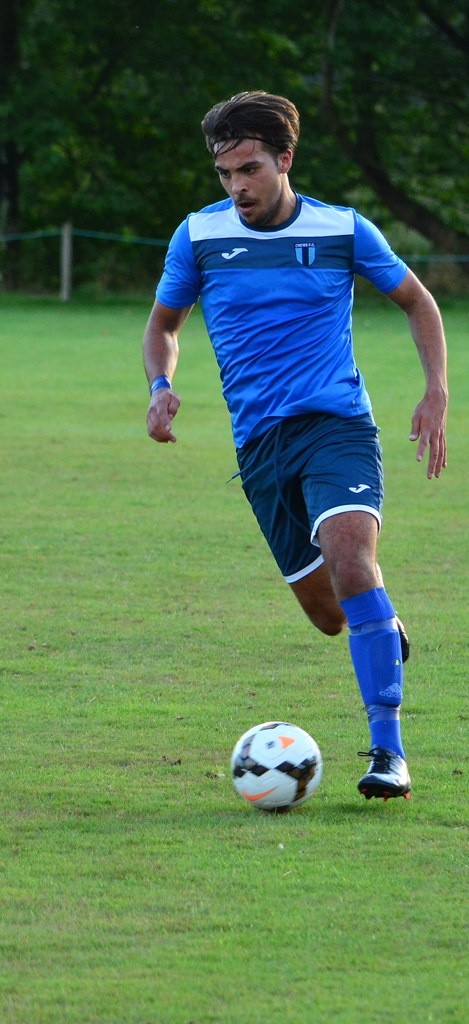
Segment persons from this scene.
[143,93,447,803]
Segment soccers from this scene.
[228,720,324,813]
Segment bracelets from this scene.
[149,375,171,396]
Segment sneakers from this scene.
[356,745,412,803]
[375,561,410,663]
